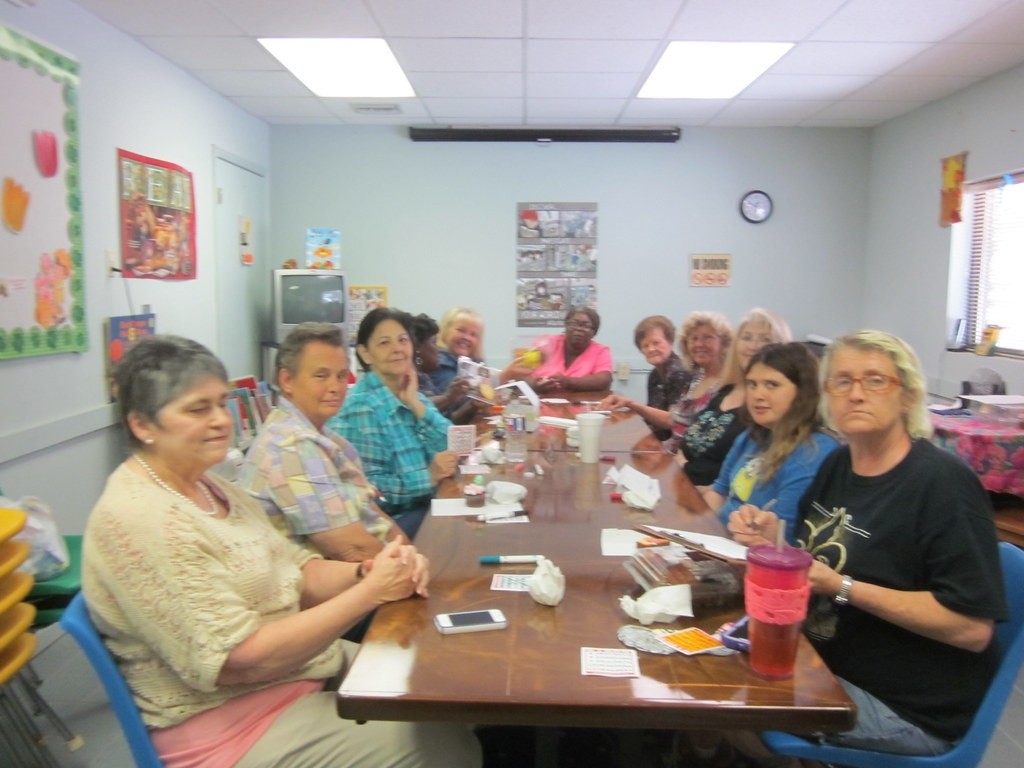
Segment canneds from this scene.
[976,325,1001,356]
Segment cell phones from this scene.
[722,614,750,652]
[433,609,507,634]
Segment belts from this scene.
[382,494,431,516]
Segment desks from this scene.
[929,403,1024,499]
[336,389,858,768]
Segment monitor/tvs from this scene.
[270,270,349,345]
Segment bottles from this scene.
[504,394,528,461]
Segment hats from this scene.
[411,313,440,344]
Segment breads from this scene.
[636,536,670,548]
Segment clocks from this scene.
[741,190,773,224]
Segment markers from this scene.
[477,510,528,522]
[572,400,600,405]
[480,555,546,563]
[611,493,661,501]
[575,452,615,461]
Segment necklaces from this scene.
[134,453,220,515]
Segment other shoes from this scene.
[654,737,735,768]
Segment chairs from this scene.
[762,540,1023,768]
[0,506,165,768]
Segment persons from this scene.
[512,304,614,393]
[702,340,843,545]
[80,332,483,768]
[592,310,733,457]
[413,313,502,425]
[236,321,410,562]
[634,314,697,440]
[322,307,461,542]
[435,306,533,392]
[675,307,793,493]
[681,328,1008,768]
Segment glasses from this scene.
[823,373,905,395]
[564,319,593,332]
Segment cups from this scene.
[744,543,813,681]
[576,412,606,464]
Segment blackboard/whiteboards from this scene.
[0,22,90,358]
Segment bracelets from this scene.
[355,563,365,584]
[831,575,852,606]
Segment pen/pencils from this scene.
[750,498,777,530]
